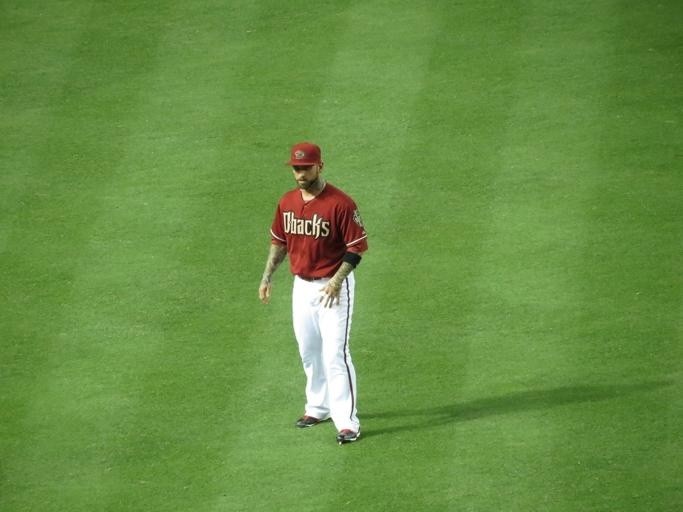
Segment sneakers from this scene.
[335,426,361,442]
[296,416,320,426]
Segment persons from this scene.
[258,141,368,445]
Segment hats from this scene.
[285,142,322,167]
